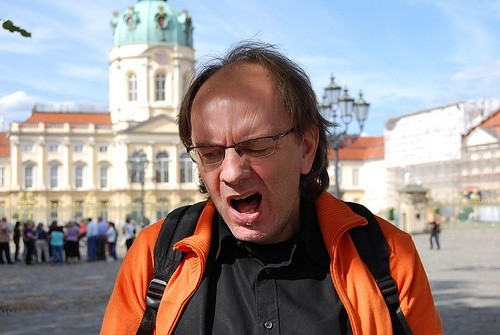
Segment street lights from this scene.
[317,74,370,201]
[126,148,149,228]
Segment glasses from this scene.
[187,125,299,167]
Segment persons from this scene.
[100,41,443,335]
[0,215,137,267]
[426,213,442,251]
